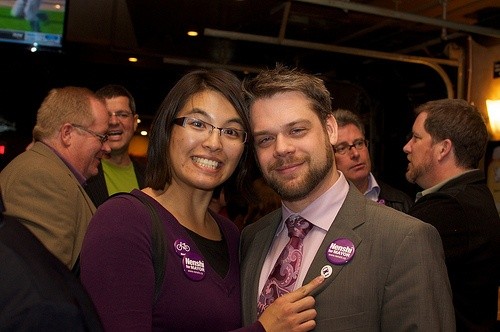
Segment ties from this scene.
[256,214,314,320]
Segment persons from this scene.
[332,98,500,332]
[237,62,456,332]
[79,70,324,332]
[0,81,279,332]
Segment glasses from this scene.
[60,123,108,144]
[334,139,369,154]
[173,116,248,144]
[108,111,134,119]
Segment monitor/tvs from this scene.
[0,0,69,51]
